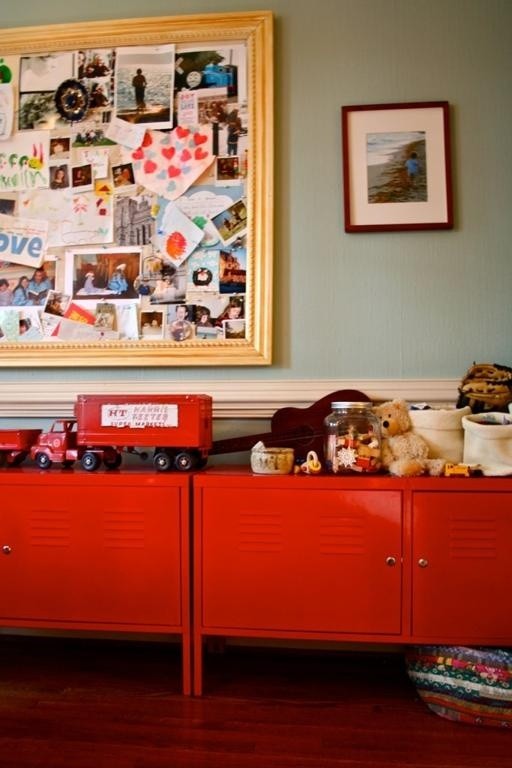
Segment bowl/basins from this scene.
[250,446,294,475]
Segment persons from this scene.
[204,100,241,155]
[142,300,245,341]
[0,267,53,336]
[405,152,419,187]
[51,169,66,190]
[79,52,147,114]
[51,140,69,160]
[74,169,86,186]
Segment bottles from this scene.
[323,401,383,474]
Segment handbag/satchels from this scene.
[403,644,512,731]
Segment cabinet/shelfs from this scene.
[1,460,512,696]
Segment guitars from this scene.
[209,389,374,463]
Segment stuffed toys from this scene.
[371,399,446,477]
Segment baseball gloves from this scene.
[458,364,511,406]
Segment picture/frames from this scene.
[0,3,280,369]
[341,97,458,234]
